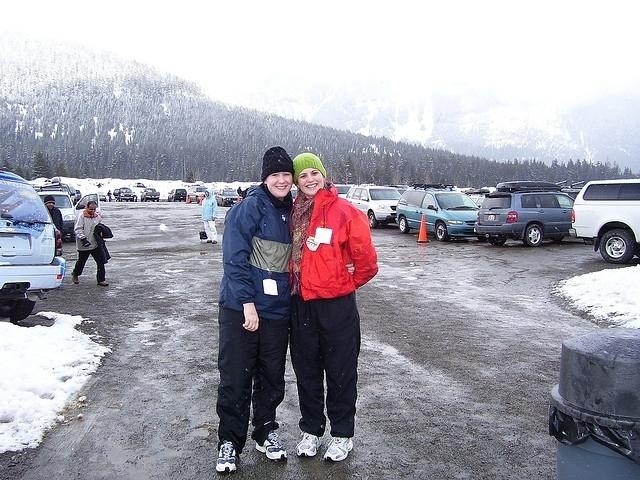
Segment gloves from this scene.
[80,238,90,247]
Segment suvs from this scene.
[0,167,107,325]
[113,187,137,201]
[568,178,640,266]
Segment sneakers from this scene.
[206,240,217,243]
[72,275,79,284]
[99,281,109,286]
[296,432,323,457]
[324,437,353,463]
[215,442,237,472]
[254,430,287,459]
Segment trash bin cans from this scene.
[548,328,640,480]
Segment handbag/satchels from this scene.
[200,232,207,238]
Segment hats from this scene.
[44,196,56,205]
[261,147,294,181]
[293,153,326,185]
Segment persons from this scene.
[71,200,110,287]
[213,146,295,474]
[288,153,379,461]
[201,187,219,244]
[43,195,63,232]
[106,189,113,202]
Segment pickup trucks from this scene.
[140,188,160,202]
